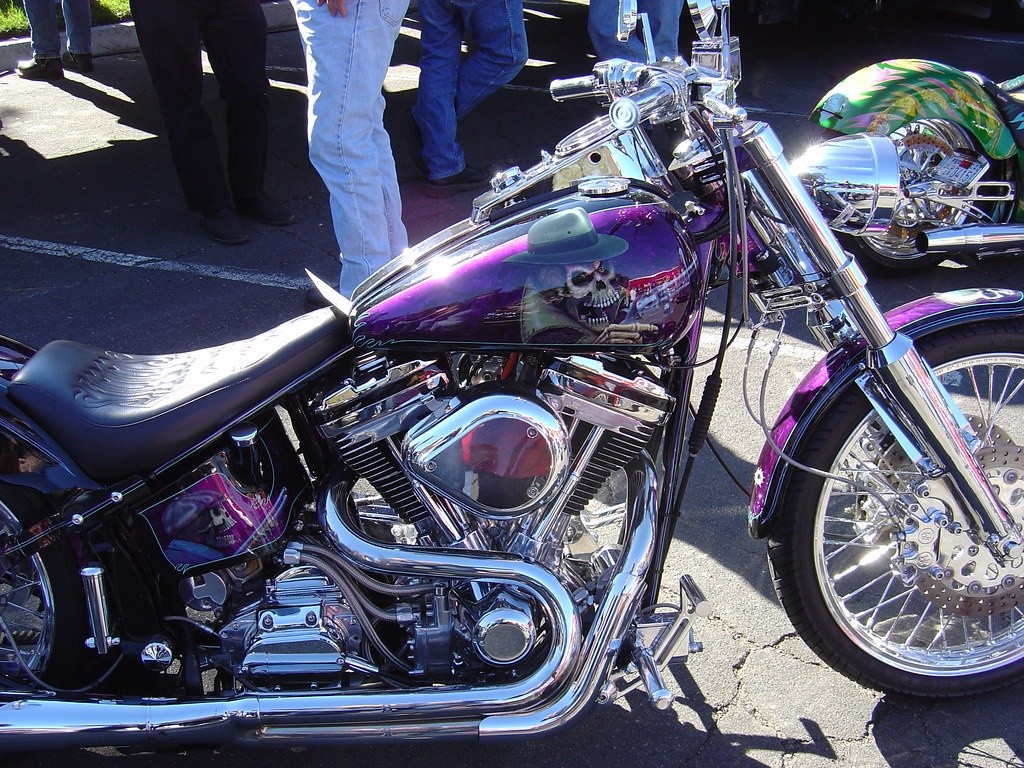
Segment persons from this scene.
[15,0,93,81]
[291,0,409,312]
[405,0,528,199]
[587,0,684,170]
[128,0,295,244]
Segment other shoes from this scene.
[234,190,296,226]
[303,285,341,310]
[200,207,250,243]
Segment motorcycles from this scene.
[1,0,1024,744]
[809,56,1024,278]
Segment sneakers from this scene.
[426,164,492,191]
[398,108,429,174]
[60,51,94,73]
[17,58,65,80]
